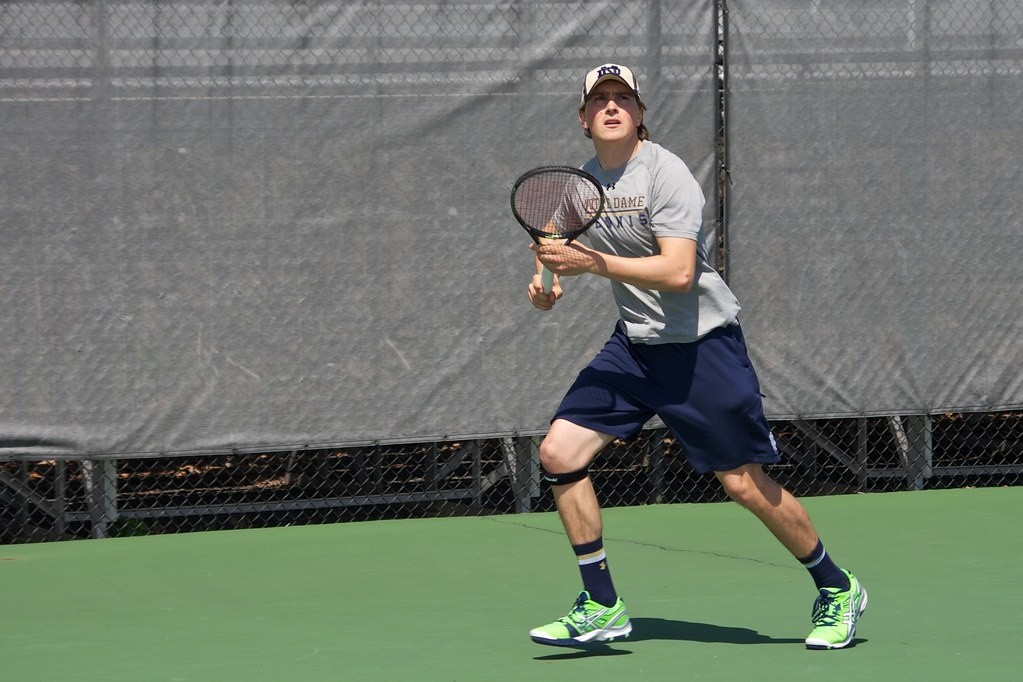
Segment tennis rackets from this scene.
[509,165,606,296]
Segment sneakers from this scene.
[805,568,868,649]
[529,590,633,647]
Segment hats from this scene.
[581,63,641,106]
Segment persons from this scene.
[529,63,868,650]
